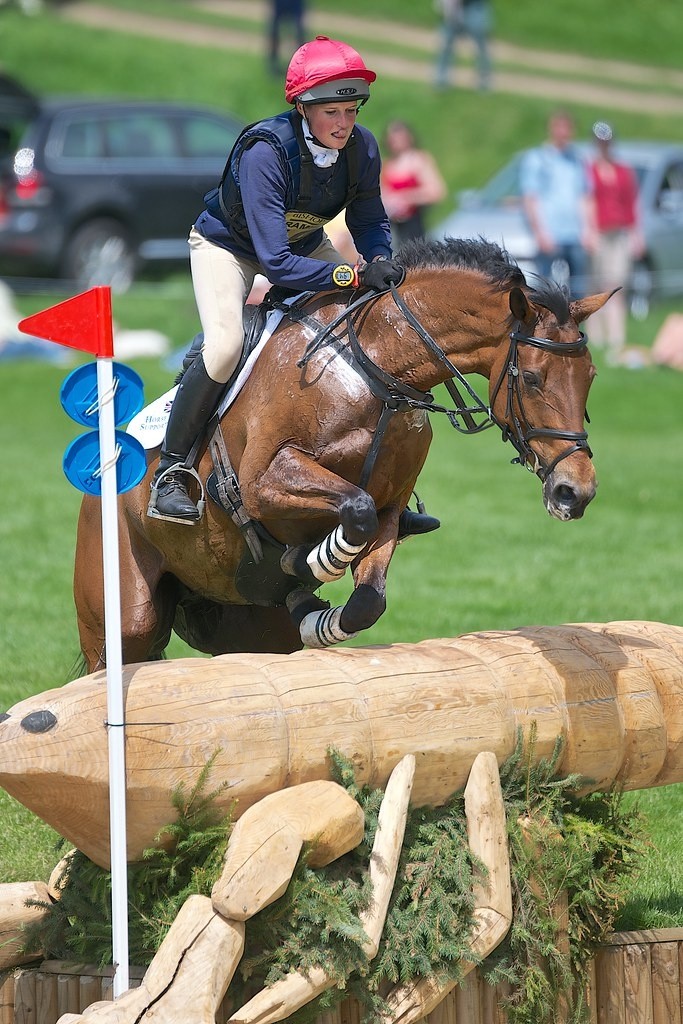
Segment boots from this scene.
[148,353,228,520]
[396,507,440,541]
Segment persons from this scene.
[263,0,306,75]
[582,122,646,353]
[148,34,405,521]
[430,0,495,101]
[518,110,594,301]
[379,119,450,264]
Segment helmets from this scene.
[285,35,377,106]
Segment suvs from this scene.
[0,72,250,299]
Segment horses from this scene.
[64,235,624,682]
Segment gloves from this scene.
[357,262,402,292]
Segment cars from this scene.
[431,141,683,304]
